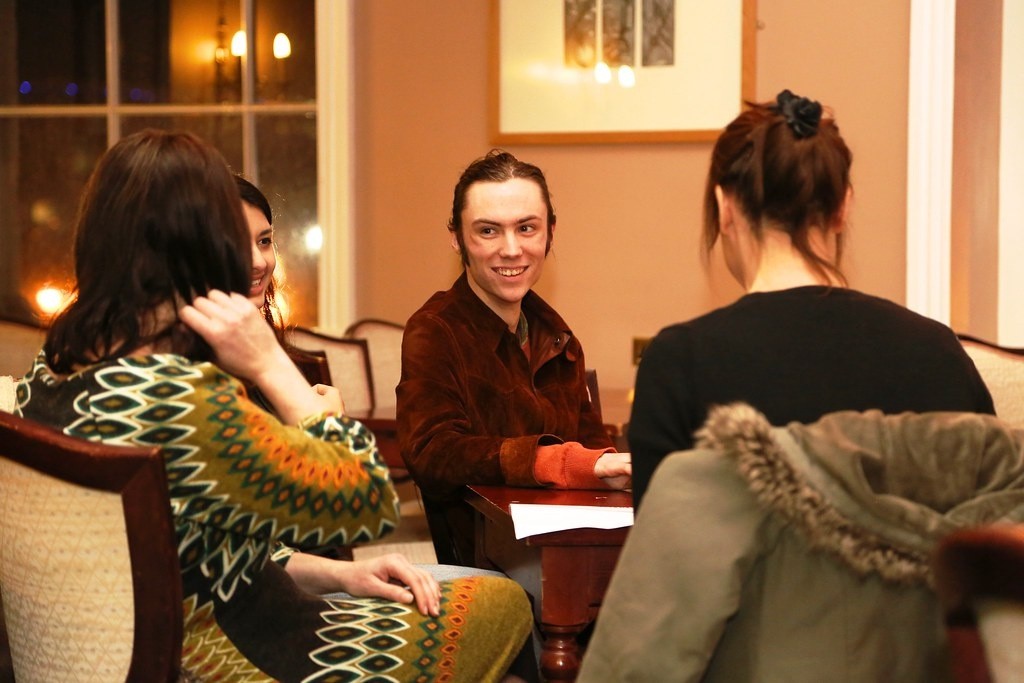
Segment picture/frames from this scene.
[485,0,757,148]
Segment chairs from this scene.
[959,331,1024,683]
[0,410,183,683]
[0,317,411,466]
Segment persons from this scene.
[628,89,997,522]
[397,149,631,674]
[17,131,539,683]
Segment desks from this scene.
[462,476,636,683]
